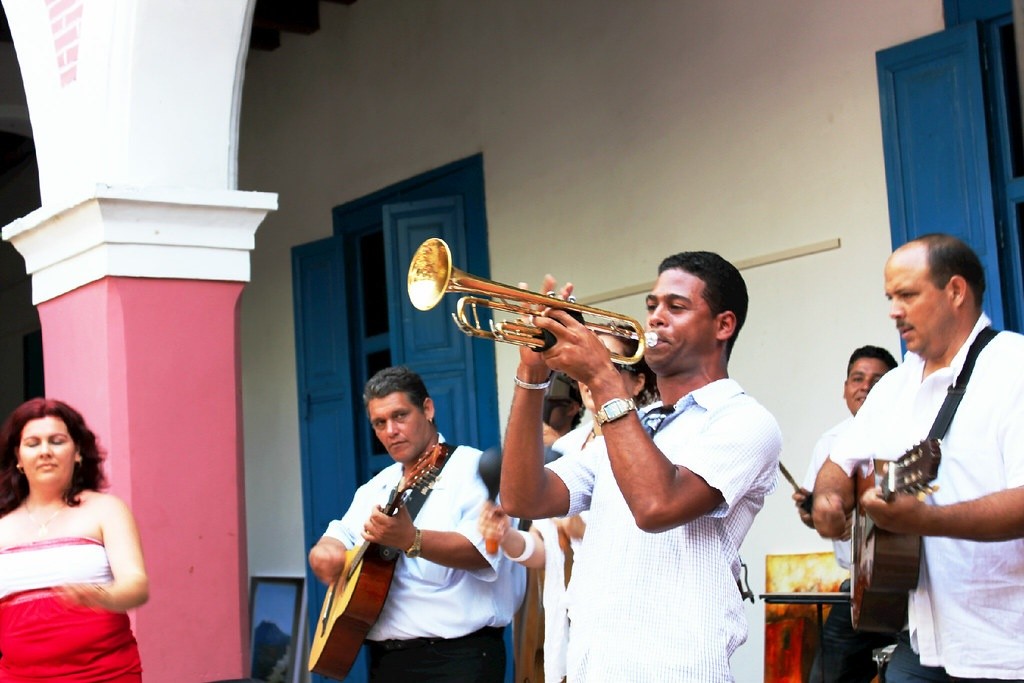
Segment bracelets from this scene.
[504,530,535,562]
[513,374,551,389]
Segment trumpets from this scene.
[405,238,657,371]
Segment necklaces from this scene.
[24,497,66,533]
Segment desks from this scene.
[759,592,851,683]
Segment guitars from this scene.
[850,436,943,631]
[309,443,443,681]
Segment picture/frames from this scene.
[250,575,308,683]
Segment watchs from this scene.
[594,397,637,425]
[405,526,421,558]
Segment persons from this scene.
[814,233,1024,683]
[309,326,659,682]
[500,251,783,682]
[792,346,900,683]
[0,395,150,683]
